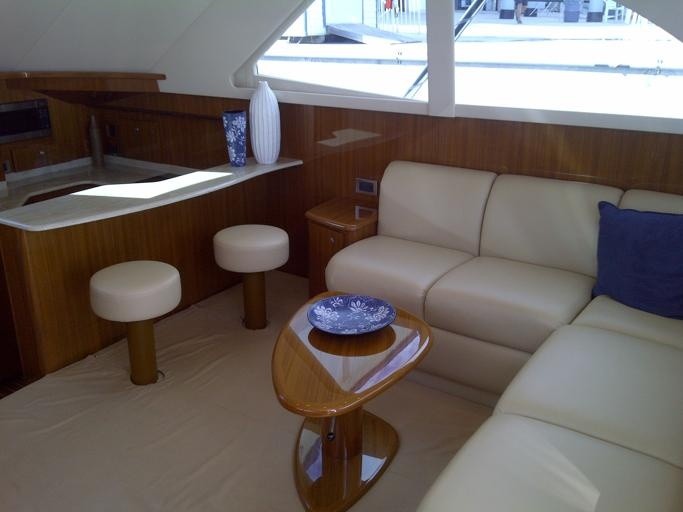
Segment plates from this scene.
[307,293,397,338]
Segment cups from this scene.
[221,109,248,167]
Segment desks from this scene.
[270,289,433,512]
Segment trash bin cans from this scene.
[499,0,515,19]
[563,0,603,22]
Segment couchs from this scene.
[323,158,683,512]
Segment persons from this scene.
[514,0,527,24]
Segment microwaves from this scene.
[0,98,54,146]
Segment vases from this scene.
[222,79,282,167]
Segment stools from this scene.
[90,225,290,386]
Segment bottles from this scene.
[86,114,110,169]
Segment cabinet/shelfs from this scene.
[303,198,377,300]
